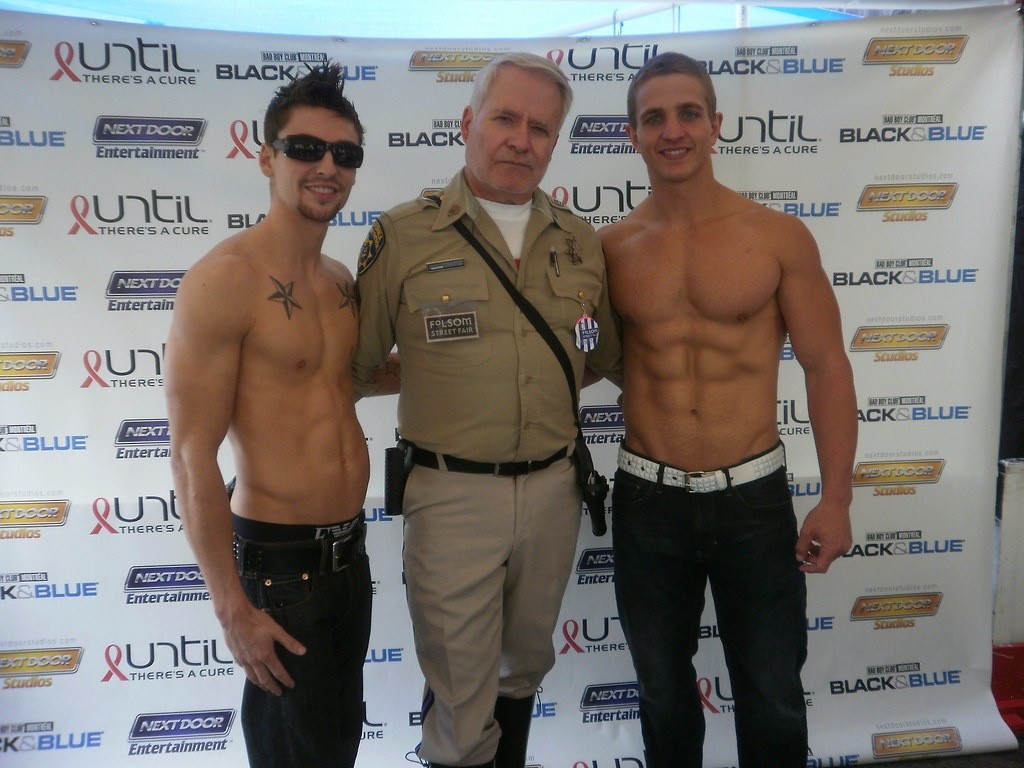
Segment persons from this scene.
[596,53,860,768]
[352,54,623,768]
[162,70,403,768]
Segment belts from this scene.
[234,524,368,572]
[407,442,568,476]
[617,446,785,493]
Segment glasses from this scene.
[272,134,363,169]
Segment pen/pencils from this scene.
[550,246,560,276]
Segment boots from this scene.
[494,694,535,768]
